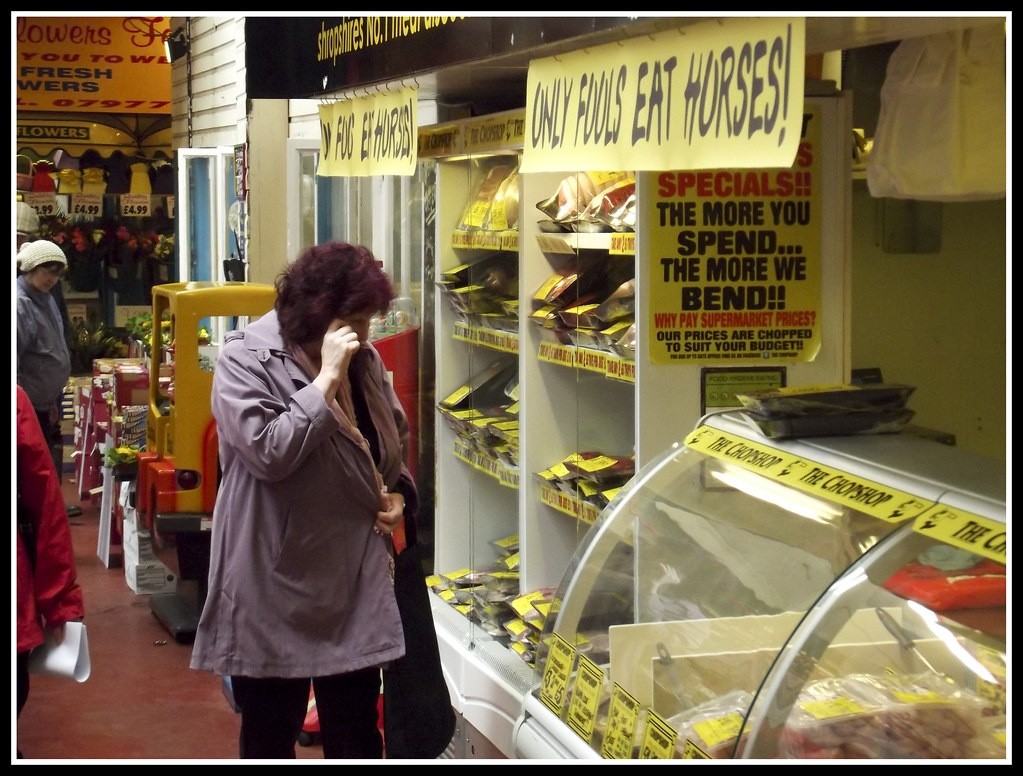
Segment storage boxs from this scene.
[62,360,177,592]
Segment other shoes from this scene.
[66,505,83,517]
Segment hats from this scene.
[17,202,39,236]
[18,240,68,272]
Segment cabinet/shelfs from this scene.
[420,88,846,760]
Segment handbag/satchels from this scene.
[382,487,456,759]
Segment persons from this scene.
[190,242,419,758]
[17,384,85,759]
[18,201,82,516]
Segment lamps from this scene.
[165,33,190,63]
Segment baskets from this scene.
[17,154,33,190]
